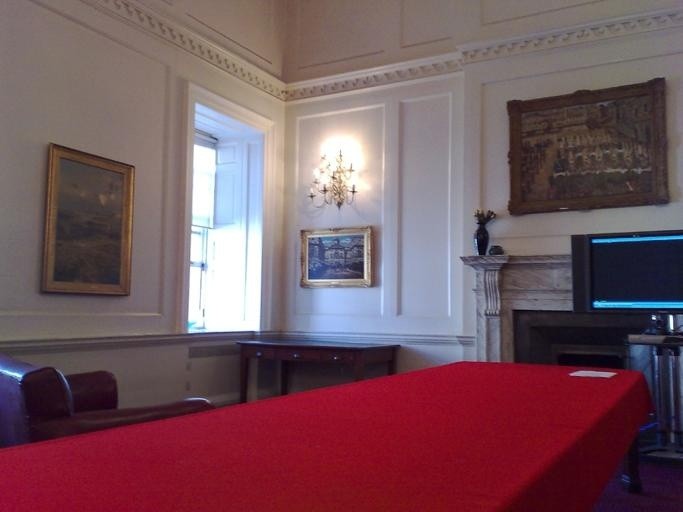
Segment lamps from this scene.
[307,149,358,209]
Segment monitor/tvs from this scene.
[570,230,683,314]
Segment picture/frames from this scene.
[301,225,374,288]
[41,141,135,296]
[504,76,669,215]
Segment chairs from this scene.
[0,353,216,449]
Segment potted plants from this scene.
[472,208,496,256]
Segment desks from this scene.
[235,337,400,403]
[0,361,651,511]
